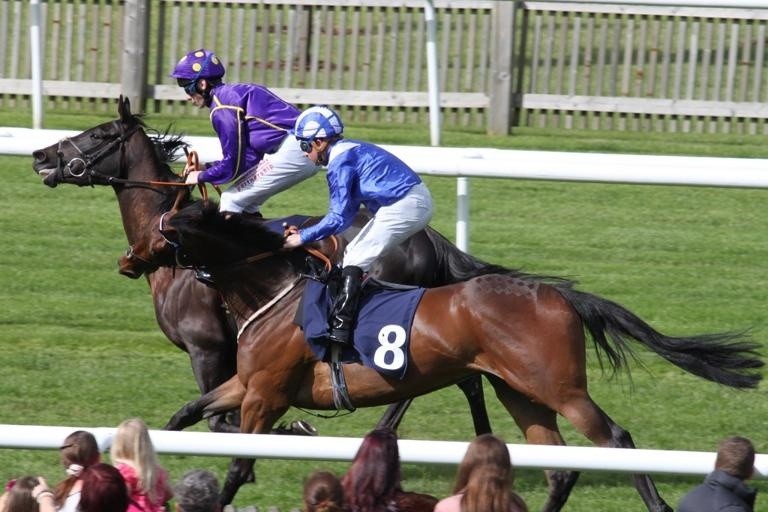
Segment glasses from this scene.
[300,139,314,153]
[184,81,197,97]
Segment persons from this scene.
[167,48,324,221]
[282,106,436,346]
[675,436,759,511]
[174,467,225,511]
[1,415,175,511]
[434,433,529,511]
[303,428,439,511]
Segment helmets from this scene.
[169,47,226,80]
[292,104,344,140]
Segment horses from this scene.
[31,93,571,440]
[117,196,768,512]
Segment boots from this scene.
[326,265,367,347]
[195,208,240,286]
